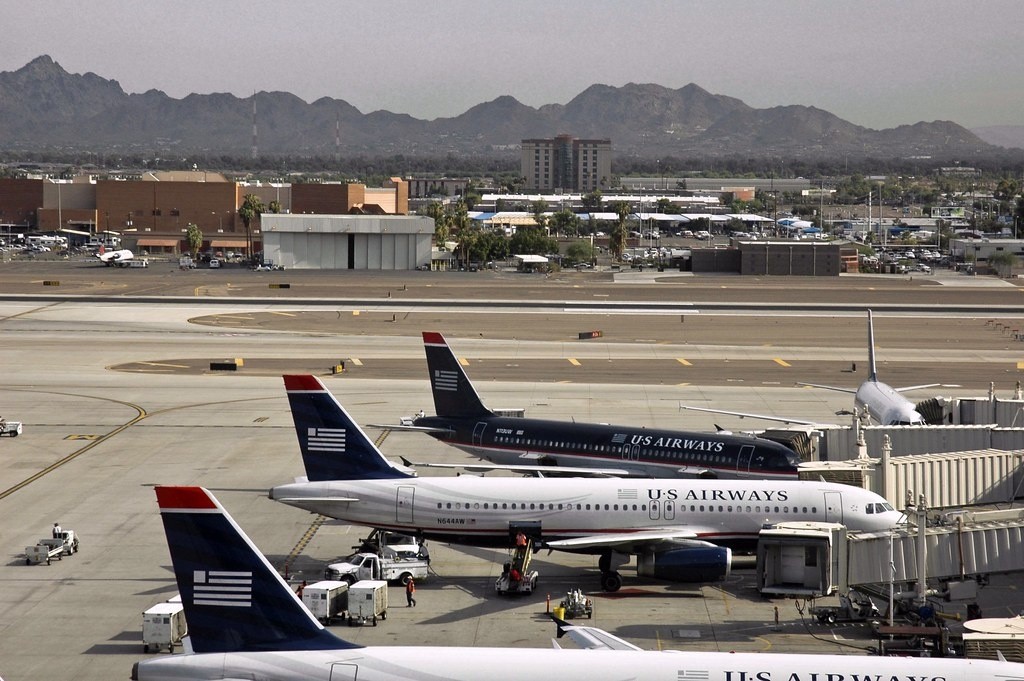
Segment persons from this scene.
[508,531,528,590]
[416,542,430,565]
[406,575,416,607]
[414,410,423,418]
[774,607,779,630]
[295,580,308,601]
[53,523,62,533]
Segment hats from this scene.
[407,576,412,580]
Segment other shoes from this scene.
[406,605,411,607]
[414,601,416,606]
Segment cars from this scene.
[0,228,263,268]
[574,222,985,274]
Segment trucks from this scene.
[321,552,429,587]
[811,590,881,627]
[25,530,79,566]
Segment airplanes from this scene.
[364,330,853,481]
[267,374,907,592]
[131,486,1024,681]
[796,308,966,428]
